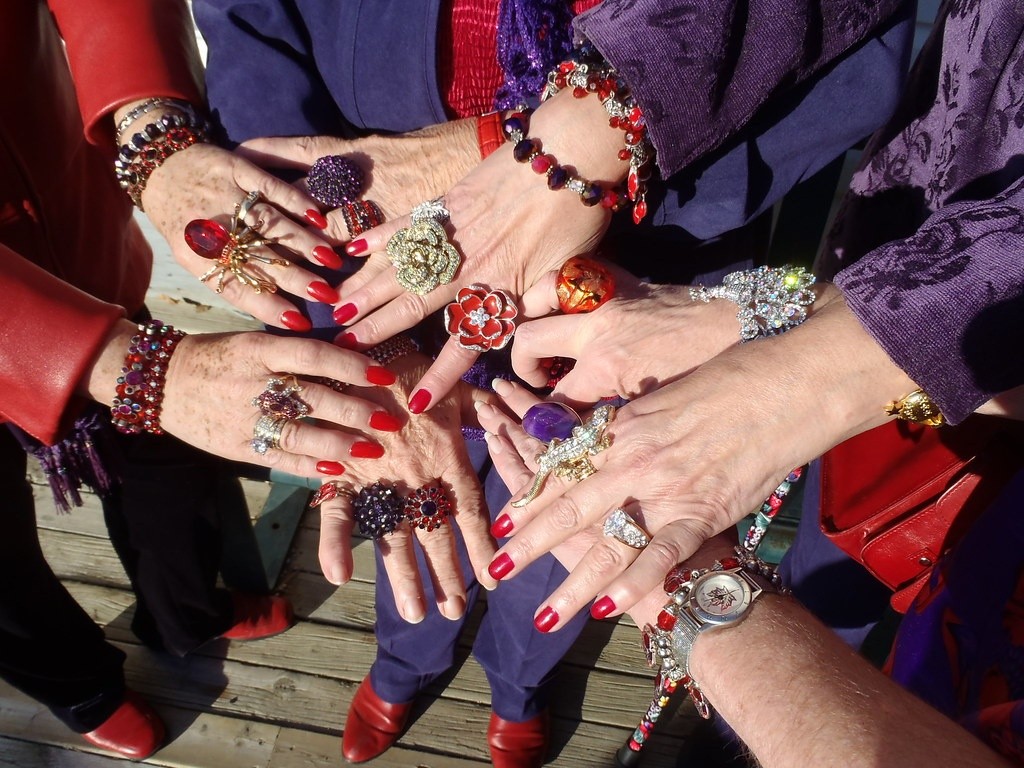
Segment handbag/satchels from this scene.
[811,408,1001,613]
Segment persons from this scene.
[0,0,404,767]
[193,0,1024,768]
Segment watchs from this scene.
[673,567,776,679]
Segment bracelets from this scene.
[690,264,819,341]
[501,105,631,213]
[885,388,940,422]
[113,98,177,150]
[115,114,193,186]
[125,130,198,212]
[320,338,419,393]
[110,320,185,433]
[640,547,783,719]
[537,57,654,187]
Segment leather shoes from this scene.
[485,706,552,768]
[83,687,172,762]
[217,594,297,642]
[340,672,414,764]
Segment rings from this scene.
[511,404,614,509]
[351,482,404,537]
[341,200,386,240]
[235,190,261,225]
[407,197,450,223]
[183,219,290,295]
[557,256,615,313]
[251,417,284,454]
[444,288,519,352]
[306,155,366,206]
[604,509,650,549]
[522,402,583,444]
[387,219,461,294]
[406,487,452,530]
[310,481,355,506]
[254,376,309,422]
